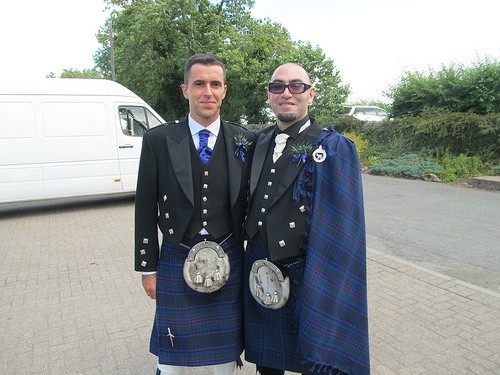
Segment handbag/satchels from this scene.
[249,258,290,309]
[183,238,230,294]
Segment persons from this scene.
[134,53,254,374]
[243,63,372,375]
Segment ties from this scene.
[196,129,213,165]
[273,119,310,163]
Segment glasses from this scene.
[268,83,312,94]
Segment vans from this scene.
[0,77,167,214]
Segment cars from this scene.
[335,106,394,121]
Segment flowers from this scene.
[233,133,253,159]
[290,143,312,168]
